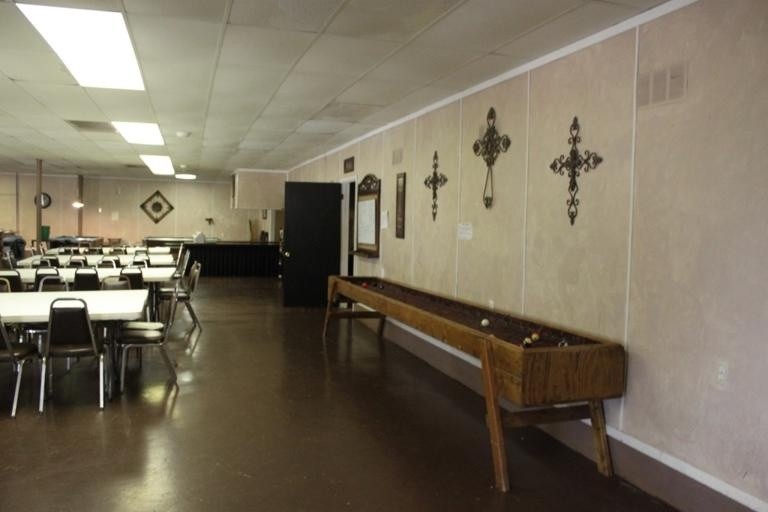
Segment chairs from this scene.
[174,250,191,278]
[134,254,150,267]
[119,266,143,289]
[35,266,60,291]
[70,255,87,266]
[64,260,84,269]
[100,275,131,357]
[175,242,184,265]
[96,258,115,268]
[158,261,197,326]
[75,268,100,289]
[41,255,60,268]
[119,284,177,370]
[116,284,179,394]
[0,316,42,418]
[7,251,17,269]
[58,240,150,254]
[161,263,203,333]
[0,277,27,375]
[1,269,21,292]
[31,258,50,267]
[24,276,71,371]
[130,260,147,268]
[102,254,120,267]
[38,298,109,412]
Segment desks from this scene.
[46,246,170,253]
[0,268,177,323]
[0,289,150,401]
[16,255,173,267]
